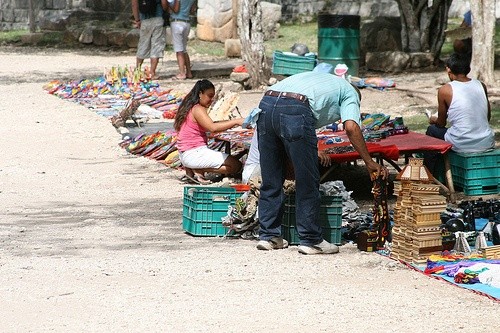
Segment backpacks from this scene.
[138,0,157,14]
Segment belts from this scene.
[169,18,190,23]
[264,91,309,105]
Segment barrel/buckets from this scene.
[317,14,361,79]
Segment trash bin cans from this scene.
[316,12,361,76]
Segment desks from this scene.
[205,132,456,204]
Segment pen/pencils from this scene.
[235,106,242,118]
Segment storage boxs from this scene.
[280,193,343,246]
[271,49,317,76]
[444,151,500,196]
[182,186,244,237]
[416,152,444,184]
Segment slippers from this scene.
[172,76,187,80]
[178,174,199,184]
[184,169,212,185]
[177,72,193,79]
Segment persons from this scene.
[167,0,196,81]
[423,53,495,179]
[132,0,167,77]
[174,79,245,186]
[256,71,389,255]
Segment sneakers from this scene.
[256,237,288,250]
[298,239,339,255]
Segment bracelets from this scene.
[136,20,141,22]
